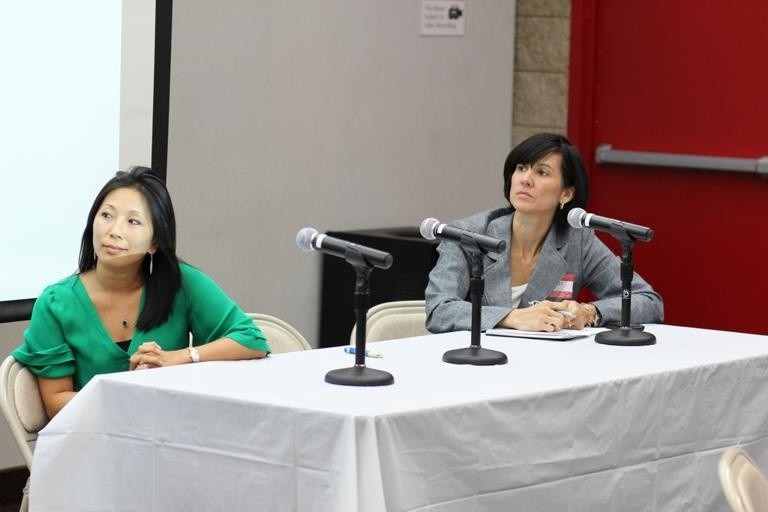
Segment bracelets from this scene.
[582,305,593,327]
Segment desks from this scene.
[57,319,767,512]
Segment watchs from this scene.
[188,347,200,363]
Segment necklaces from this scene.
[122,320,129,328]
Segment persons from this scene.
[424,132,665,335]
[10,165,271,421]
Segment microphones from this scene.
[567,206,654,243]
[420,216,506,255]
[295,227,393,271]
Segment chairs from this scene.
[0,352,50,512]
[189,312,314,356]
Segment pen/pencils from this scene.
[529,301,576,320]
[345,348,380,361]
